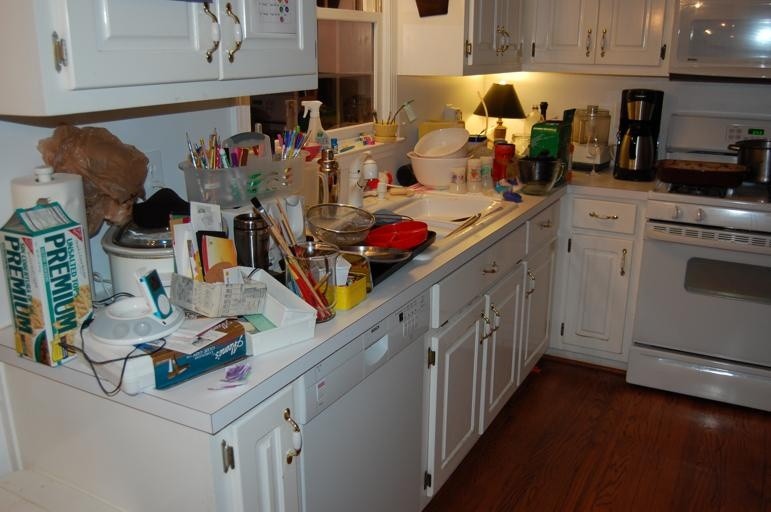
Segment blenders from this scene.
[569,104,612,174]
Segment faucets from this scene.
[348,151,379,205]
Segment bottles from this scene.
[523,104,545,135]
[232,213,270,271]
[448,156,493,193]
[317,149,340,218]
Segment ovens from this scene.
[629,219,771,373]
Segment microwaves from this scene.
[666,0,770,85]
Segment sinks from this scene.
[396,198,517,225]
[334,218,460,267]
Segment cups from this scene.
[285,242,340,323]
[374,120,399,137]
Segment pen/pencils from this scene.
[250,196,332,321]
[278,126,311,160]
[186,128,249,203]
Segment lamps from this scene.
[470,82,528,147]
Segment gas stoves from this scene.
[643,164,771,235]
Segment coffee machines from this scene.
[613,89,665,182]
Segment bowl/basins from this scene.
[514,158,566,196]
[365,221,428,252]
[407,151,469,191]
[302,144,322,162]
[413,127,470,158]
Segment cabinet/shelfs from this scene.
[0,1,318,120]
[425,222,528,510]
[546,190,645,374]
[525,1,680,79]
[395,0,525,80]
[519,197,557,390]
[216,386,304,511]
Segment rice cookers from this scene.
[101,217,176,304]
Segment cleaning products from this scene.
[300,100,328,143]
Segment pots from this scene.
[726,137,769,185]
